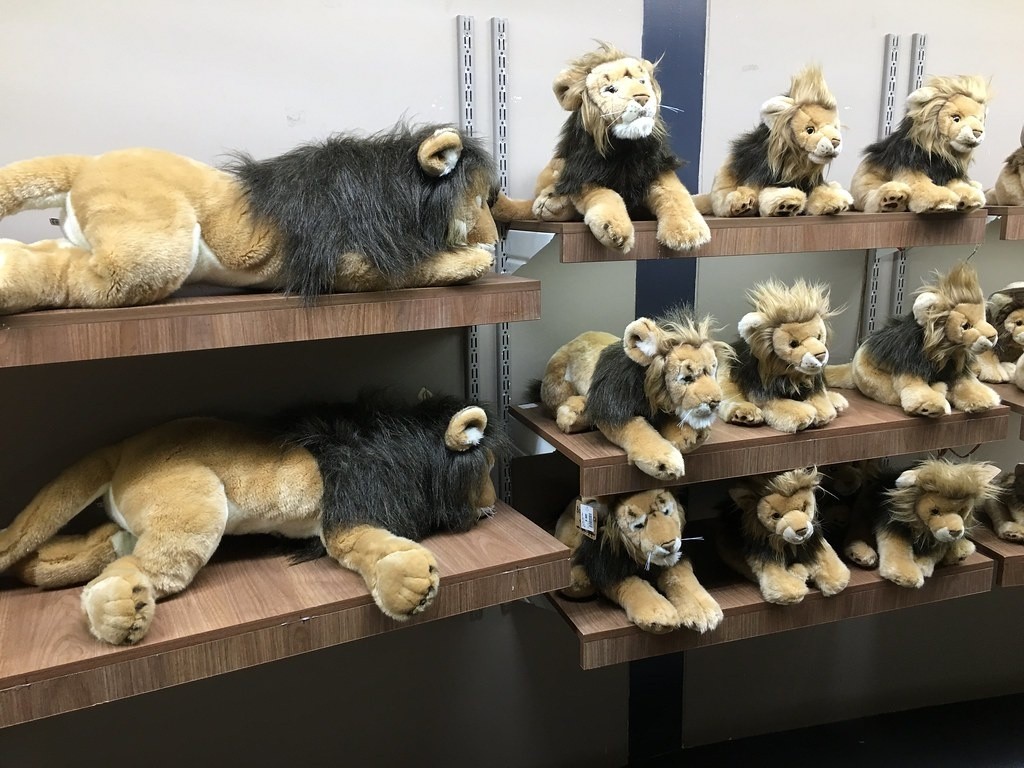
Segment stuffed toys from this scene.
[0,392,515,646]
[491,39,1023,633]
[0,120,503,313]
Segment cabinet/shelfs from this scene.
[0,204,1024,735]
